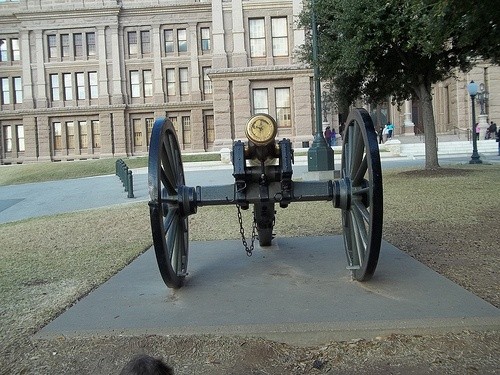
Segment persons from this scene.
[486,122,500,156]
[325,126,331,148]
[331,128,336,145]
[475,123,481,141]
[377,122,394,144]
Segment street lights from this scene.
[467,80,482,163]
[476,83,489,114]
